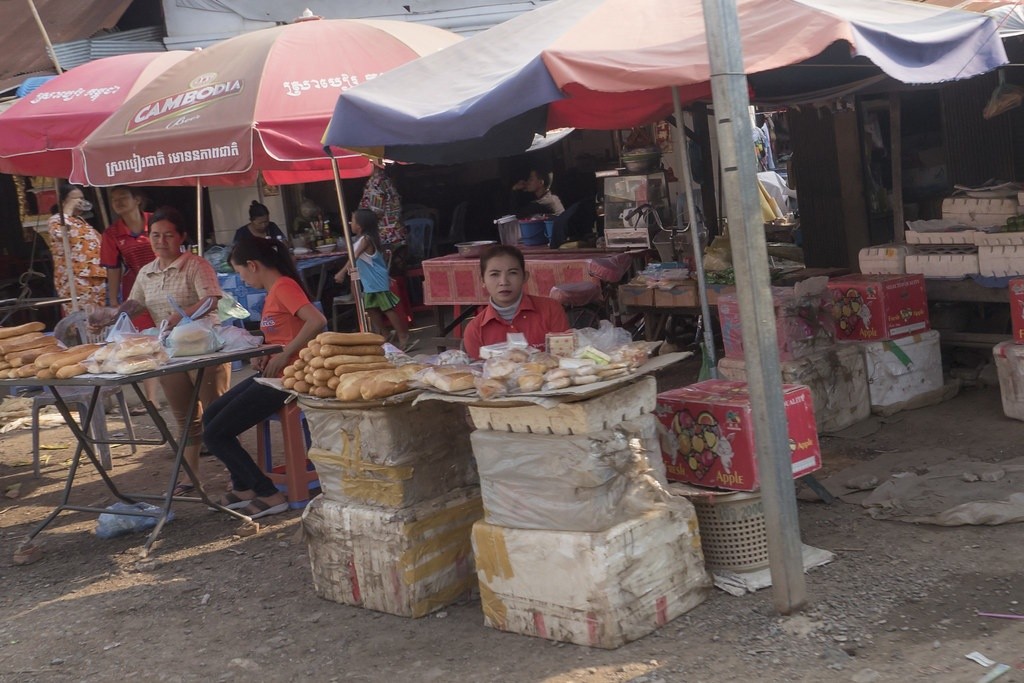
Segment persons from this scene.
[358,167,416,328]
[462,244,572,362]
[507,168,565,217]
[99,185,165,415]
[334,208,421,353]
[201,236,328,518]
[47,183,107,337]
[87,205,232,496]
[756,111,775,170]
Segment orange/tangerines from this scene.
[999,215,1024,232]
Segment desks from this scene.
[215,251,350,340]
[0,342,284,558]
[422,251,631,350]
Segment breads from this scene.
[0,322,212,378]
[282,332,649,400]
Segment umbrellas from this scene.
[0,47,206,259]
[69,9,465,333]
[321,1,1008,379]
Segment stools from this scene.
[403,268,437,318]
[376,275,414,329]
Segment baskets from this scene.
[691,481,769,573]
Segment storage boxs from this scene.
[718,191,1024,436]
[302,376,710,651]
[659,376,823,493]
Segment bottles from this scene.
[81,200,93,211]
[315,229,324,252]
[294,246,312,255]
[323,221,334,244]
[192,245,198,256]
[316,228,324,246]
[337,235,345,252]
[277,235,283,243]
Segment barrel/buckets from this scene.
[518,221,549,246]
[543,220,555,244]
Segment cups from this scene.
[497,215,518,247]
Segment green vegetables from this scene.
[704,268,735,285]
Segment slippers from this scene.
[397,338,420,353]
[200,436,240,456]
[231,498,290,520]
[384,330,397,343]
[173,481,204,497]
[208,490,252,511]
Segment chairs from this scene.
[333,248,391,333]
[401,216,434,268]
[32,306,138,478]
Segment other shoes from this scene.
[130,399,163,416]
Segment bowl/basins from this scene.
[316,244,335,254]
[622,152,664,173]
[454,241,499,258]
